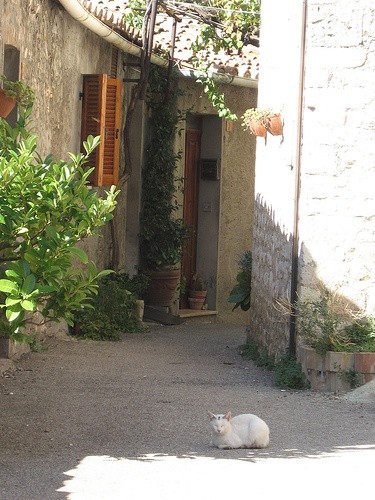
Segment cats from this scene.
[205,409,270,450]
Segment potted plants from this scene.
[293,290,353,392]
[345,313,375,388]
[119,273,150,321]
[137,213,193,307]
[239,107,283,137]
[0,75,35,118]
[186,271,208,310]
